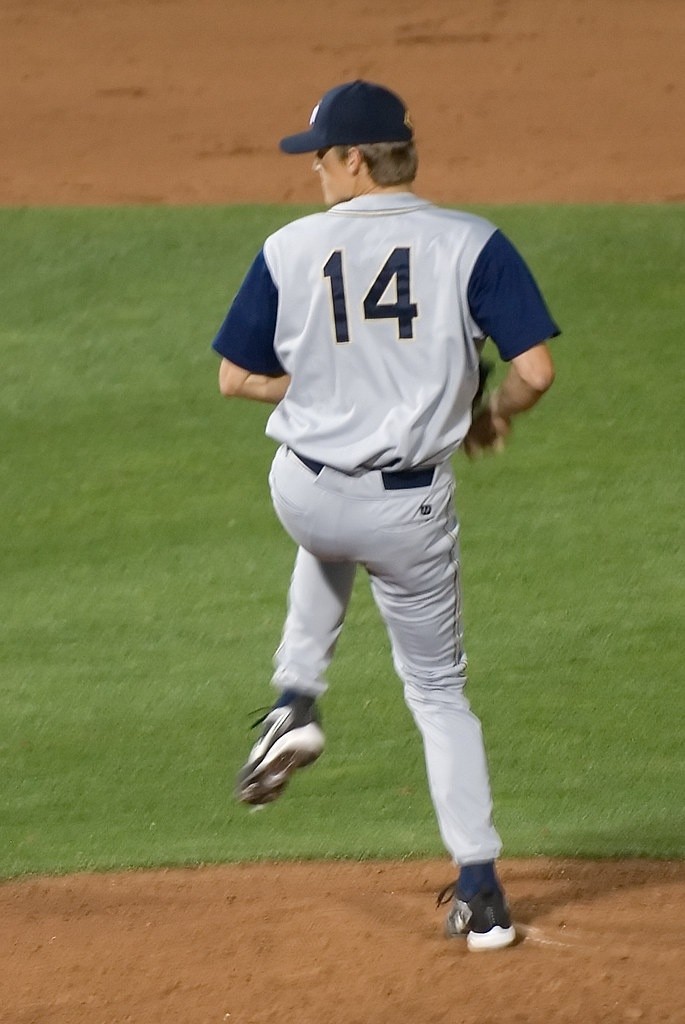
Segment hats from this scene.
[279,79,412,154]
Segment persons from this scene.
[212,80,562,952]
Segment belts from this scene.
[288,448,436,490]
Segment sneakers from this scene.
[435,876,516,954]
[238,696,325,804]
